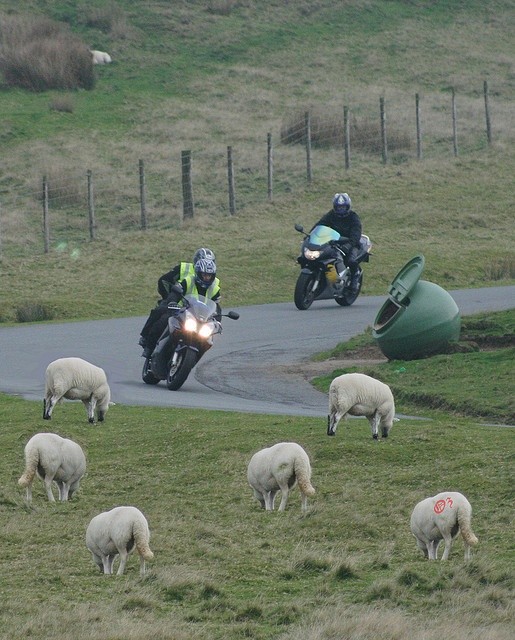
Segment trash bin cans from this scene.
[372,255,461,360]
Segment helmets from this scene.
[333,193,352,218]
[194,247,215,259]
[194,259,217,289]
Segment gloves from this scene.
[341,244,353,254]
[167,301,181,312]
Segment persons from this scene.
[137,247,216,346]
[303,191,360,292]
[141,259,221,359]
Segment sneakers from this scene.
[139,337,146,347]
[141,342,155,358]
[350,279,360,293]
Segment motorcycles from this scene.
[294,224,374,310]
[141,284,240,390]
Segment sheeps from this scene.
[18,432,88,503]
[326,372,404,438]
[409,491,481,563]
[43,356,116,424]
[245,442,316,514]
[84,505,154,576]
